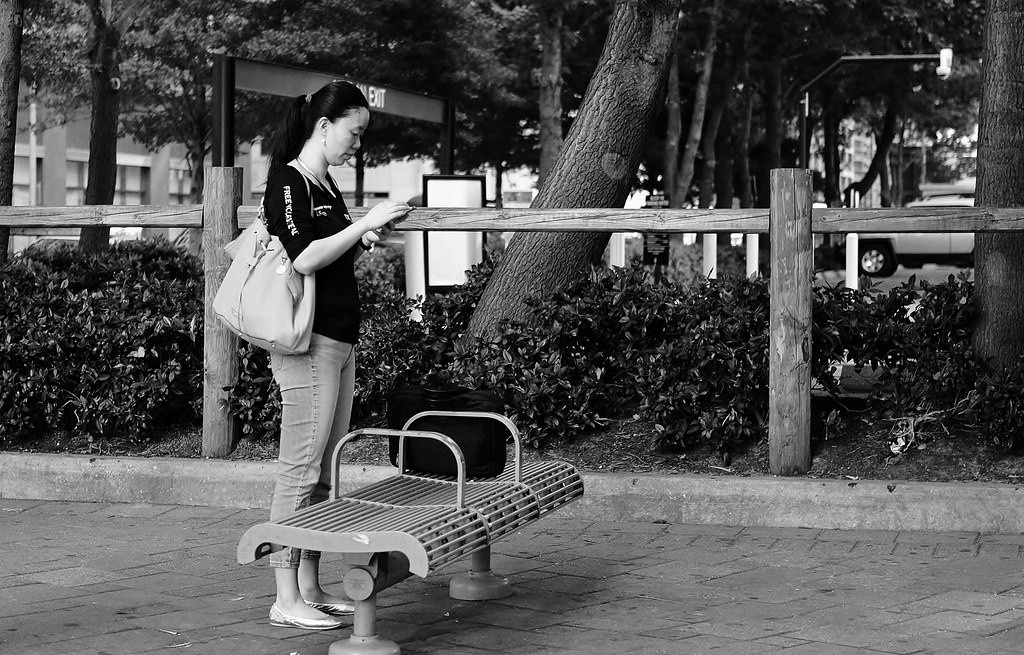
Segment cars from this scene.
[833,195,974,279]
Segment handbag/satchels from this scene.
[211,164,315,355]
[385,382,506,479]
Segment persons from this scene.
[266,81,410,631]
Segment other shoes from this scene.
[268,602,343,629]
[303,599,356,615]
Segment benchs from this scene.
[236,411,585,655]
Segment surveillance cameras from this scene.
[936,66,948,77]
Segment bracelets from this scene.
[358,237,372,251]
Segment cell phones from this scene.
[392,206,417,223]
